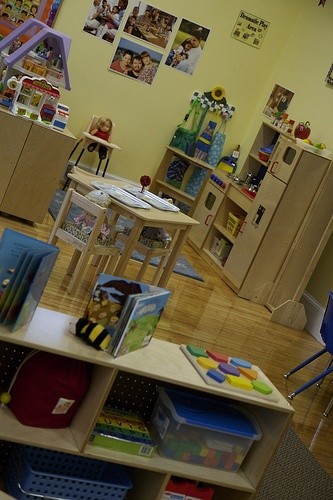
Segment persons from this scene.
[84,0,202,84]
[273,91,289,115]
[88,118,112,159]
[74,190,113,241]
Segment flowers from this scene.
[211,85,226,100]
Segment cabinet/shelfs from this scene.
[0,105,77,223]
[0,307,293,500]
[148,121,333,330]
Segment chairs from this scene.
[283,291,333,416]
[95,228,181,287]
[63,115,121,191]
[47,188,120,289]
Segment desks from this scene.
[67,173,201,289]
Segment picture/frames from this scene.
[324,64,333,85]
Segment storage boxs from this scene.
[138,382,262,473]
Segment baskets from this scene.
[1,443,132,500]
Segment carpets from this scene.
[256,427,333,500]
[49,190,207,283]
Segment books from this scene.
[85,273,171,359]
[0,229,60,333]
